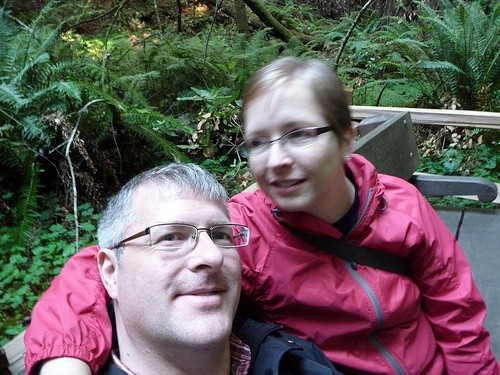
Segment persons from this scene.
[24,56,500,375]
[90,163,342,375]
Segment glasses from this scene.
[111,223,250,250]
[237,125,334,158]
